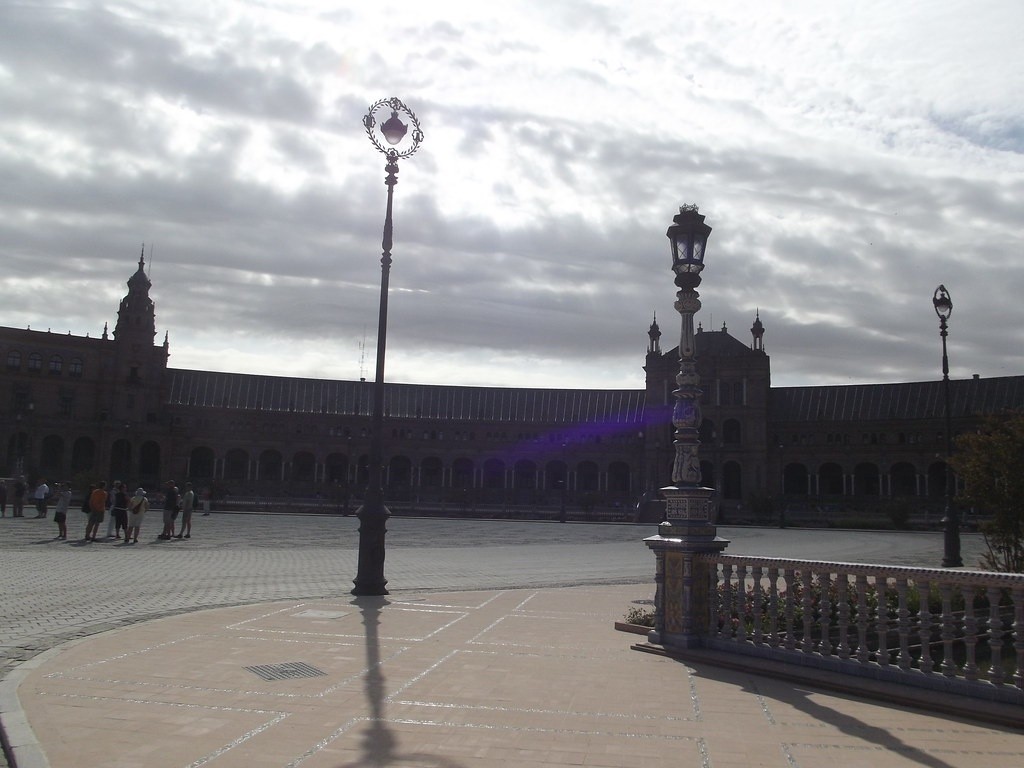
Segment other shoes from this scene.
[158,534,171,540]
[134,539,138,543]
[56,534,66,540]
[91,537,98,541]
[85,534,90,540]
[184,534,190,538]
[174,534,183,538]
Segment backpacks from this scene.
[192,489,200,508]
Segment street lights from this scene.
[663,204,713,485]
[930,283,965,570]
[350,95,424,594]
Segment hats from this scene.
[134,487,147,496]
[165,479,176,485]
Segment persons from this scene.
[0,480,7,517]
[53,480,73,540]
[81,478,211,543]
[32,479,50,519]
[13,474,29,517]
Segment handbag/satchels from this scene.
[132,505,140,514]
[111,508,117,516]
[82,502,90,513]
